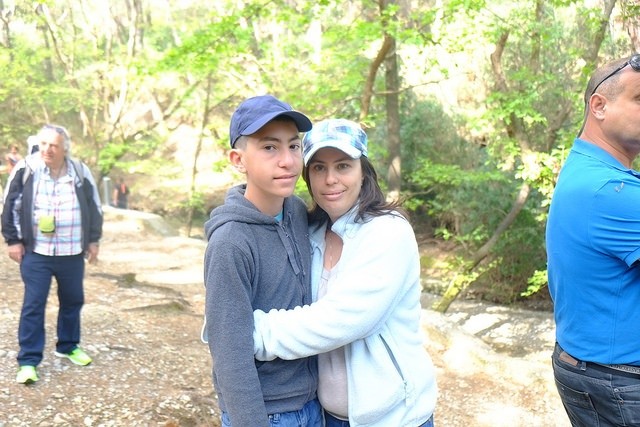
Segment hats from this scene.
[227,95,312,149]
[302,116,368,168]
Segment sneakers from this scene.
[16,365,39,384]
[53,346,93,366]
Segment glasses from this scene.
[588,54,640,95]
[44,124,66,134]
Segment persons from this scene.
[546,54,640,427]
[0,125,103,384]
[200,118,439,427]
[4,143,22,177]
[203,96,326,427]
[110,176,130,208]
